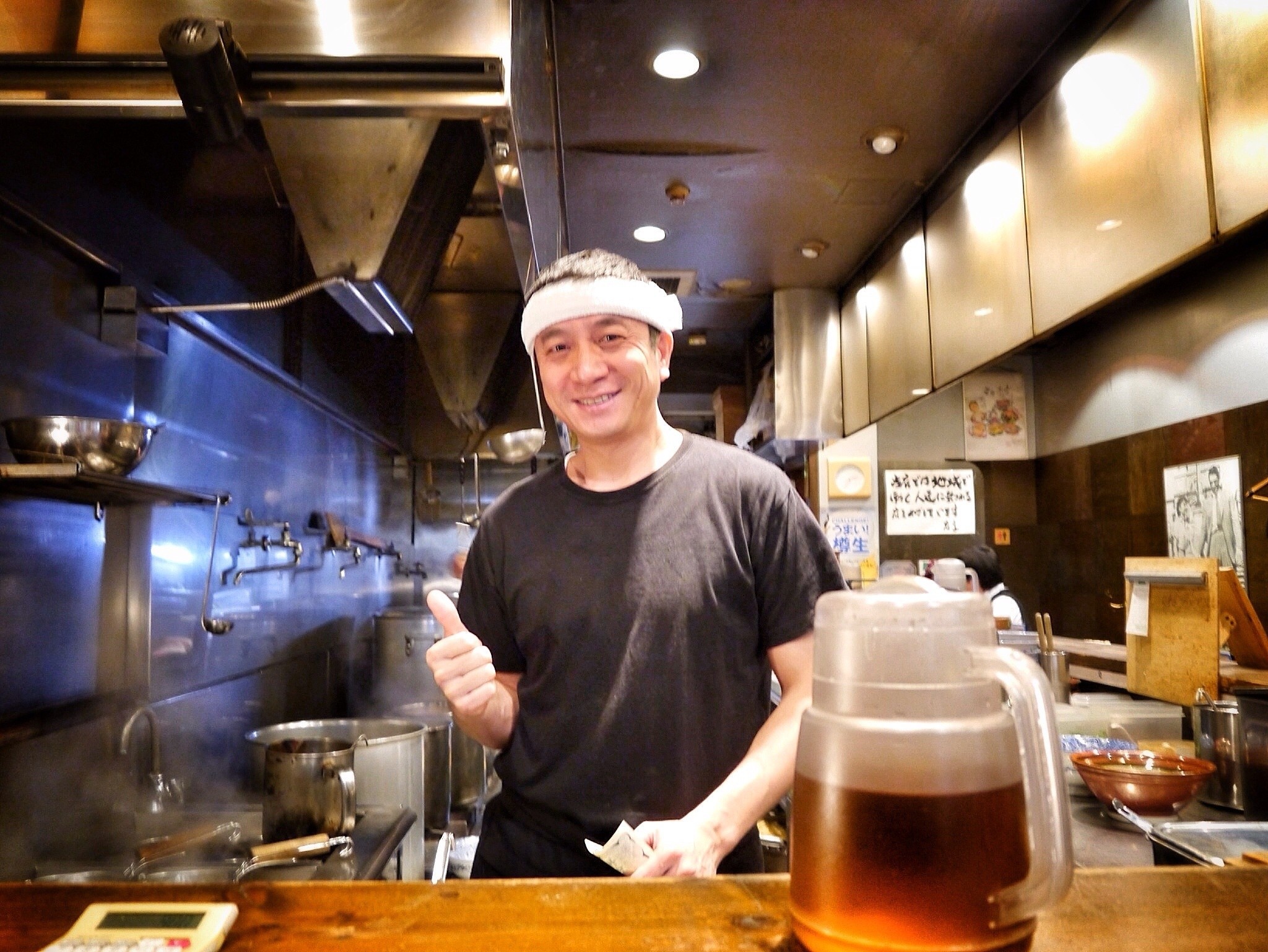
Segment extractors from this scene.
[402,181,536,463]
[253,94,487,342]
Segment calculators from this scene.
[37,901,239,952]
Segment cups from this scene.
[258,733,362,840]
[1034,647,1073,705]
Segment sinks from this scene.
[124,854,326,885]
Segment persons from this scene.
[956,544,1028,631]
[425,249,850,882]
[1171,465,1243,570]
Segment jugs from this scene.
[783,573,1077,951]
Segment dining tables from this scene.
[0,859,1268,952]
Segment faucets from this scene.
[394,561,428,579]
[236,507,303,586]
[114,704,185,835]
[321,525,362,579]
[381,541,404,560]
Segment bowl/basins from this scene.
[1071,746,1217,825]
[5,405,163,479]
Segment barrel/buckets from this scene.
[246,709,429,894]
[371,594,493,830]
